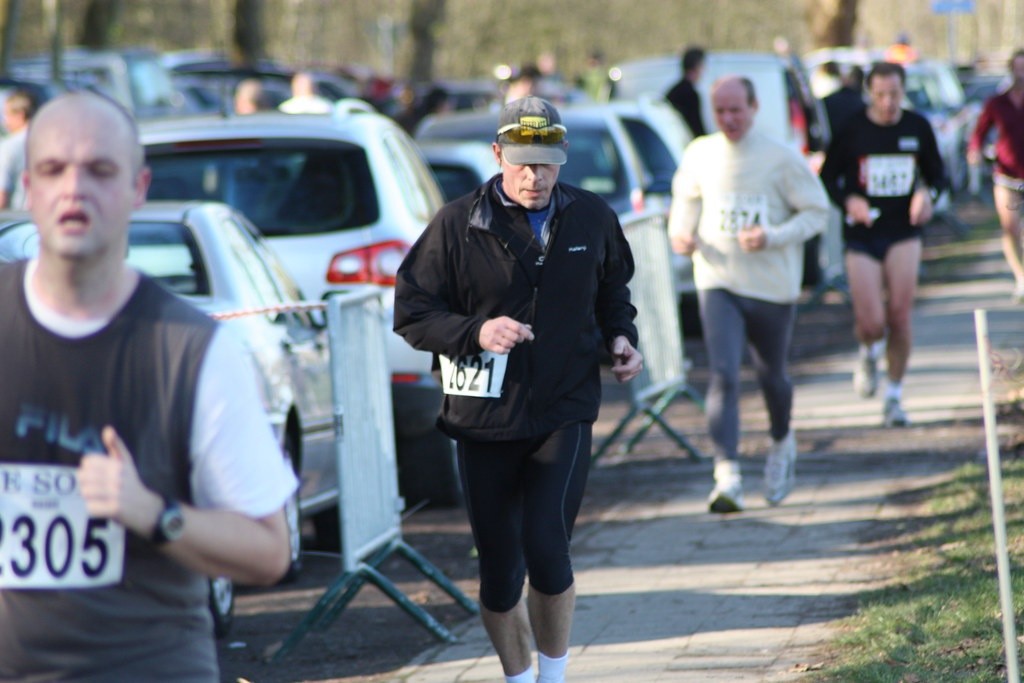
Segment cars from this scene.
[7,50,699,332]
[0,202,340,635]
[604,42,966,289]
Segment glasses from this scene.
[496,123,567,146]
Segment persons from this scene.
[234,79,270,113]
[968,49,1024,303]
[820,62,862,133]
[666,74,832,513]
[278,70,334,114]
[842,66,864,91]
[819,62,945,428]
[670,48,709,137]
[0,85,300,683]
[393,94,645,683]
[0,90,40,211]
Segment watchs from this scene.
[149,497,184,547]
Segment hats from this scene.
[495,94,568,165]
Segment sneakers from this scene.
[851,352,878,398]
[883,397,906,428]
[708,464,744,512]
[765,429,796,505]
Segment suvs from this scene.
[13,99,462,506]
